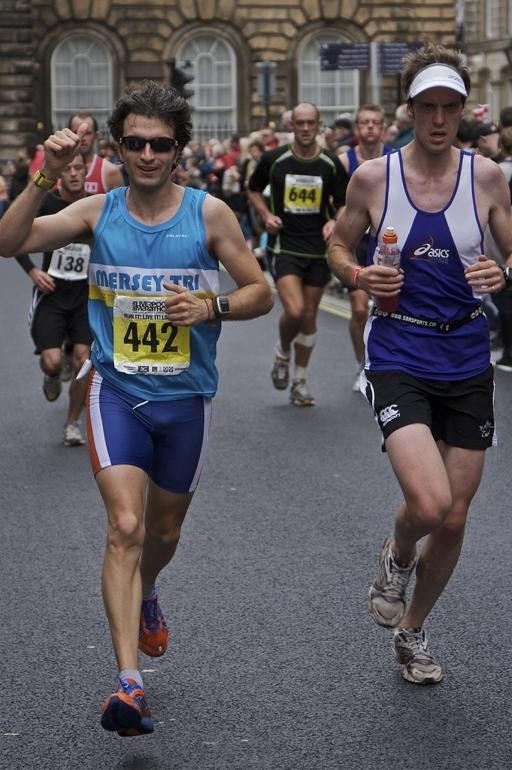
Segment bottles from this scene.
[373,226,401,316]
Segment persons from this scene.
[325,42,510,685]
[316,101,511,366]
[171,110,301,278]
[4,112,131,211]
[2,74,278,738]
[250,99,351,408]
[9,150,104,449]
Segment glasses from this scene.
[119,136,181,151]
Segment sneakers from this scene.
[389,626,444,684]
[369,537,417,628]
[272,361,288,389]
[62,424,85,446]
[61,337,76,380]
[139,588,168,656]
[42,374,61,401]
[101,675,154,737]
[289,380,315,406]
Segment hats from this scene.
[469,122,499,147]
[406,62,468,100]
